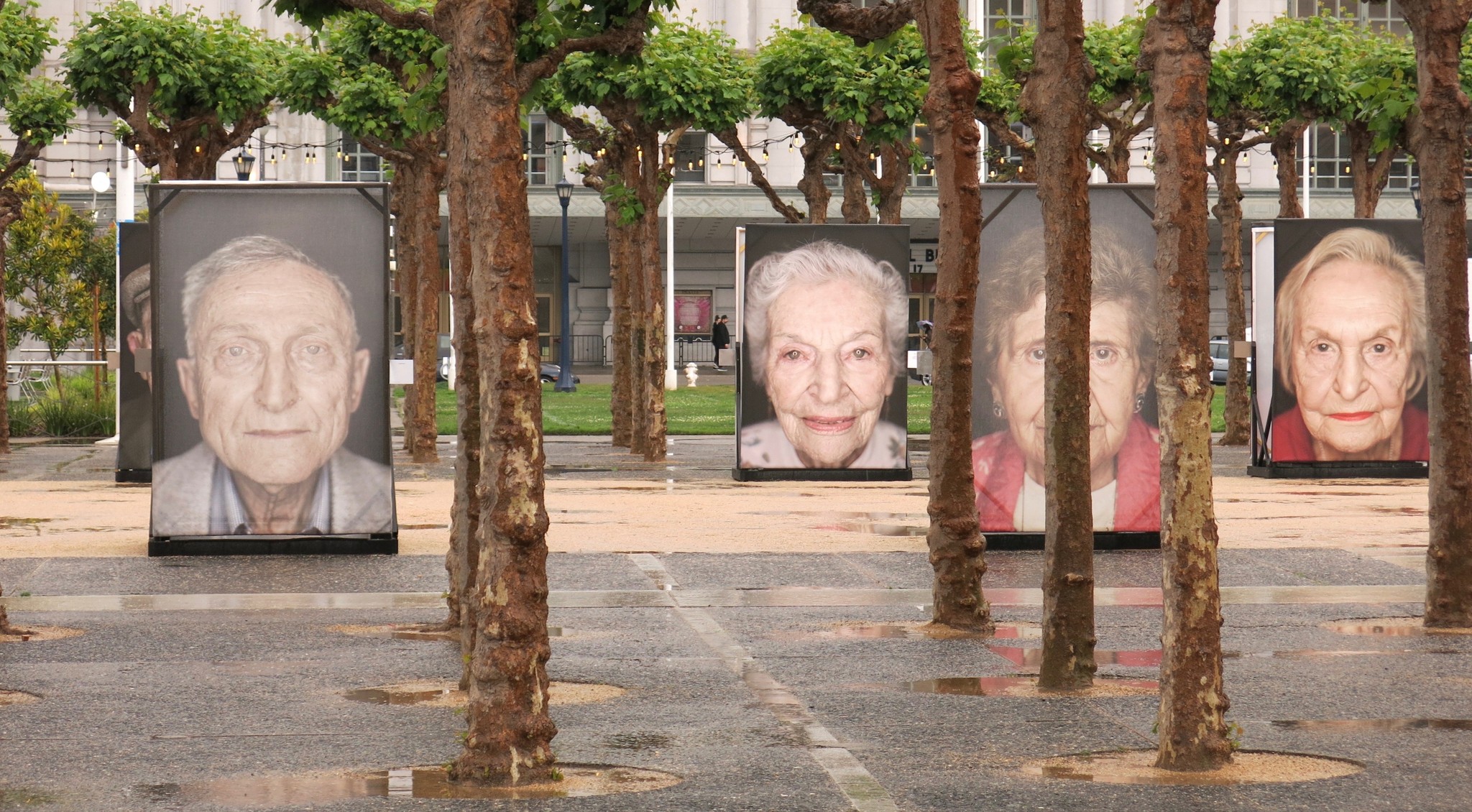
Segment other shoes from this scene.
[718,368,727,371]
[713,362,719,368]
[713,368,718,371]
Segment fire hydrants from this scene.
[682,362,699,387]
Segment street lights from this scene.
[553,172,577,393]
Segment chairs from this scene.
[6,357,54,402]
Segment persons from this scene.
[116,262,152,469]
[152,237,392,537]
[738,240,909,470]
[1270,228,1429,461]
[711,314,730,372]
[918,324,932,351]
[969,213,1163,532]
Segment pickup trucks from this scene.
[907,349,933,386]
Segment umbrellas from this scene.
[916,320,933,331]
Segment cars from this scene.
[393,332,582,383]
[1209,336,1252,388]
